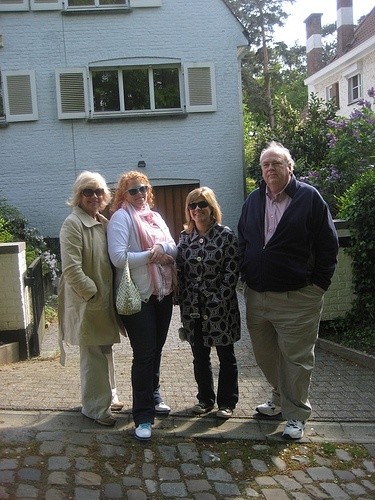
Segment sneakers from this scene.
[256,399,282,416]
[282,419,305,439]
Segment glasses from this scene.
[125,186,148,195]
[188,201,209,210]
[80,188,104,197]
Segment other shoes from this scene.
[154,401,171,413]
[193,403,213,413]
[217,407,233,417]
[111,400,124,410]
[134,423,152,440]
[95,414,116,425]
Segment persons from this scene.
[107,171,175,441]
[176,187,240,418]
[57,170,127,426]
[236,142,340,439]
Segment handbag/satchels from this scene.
[113,251,141,316]
